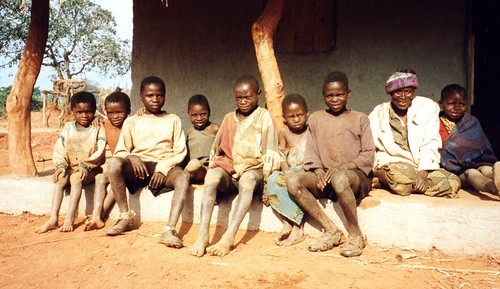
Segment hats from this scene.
[385,68,418,94]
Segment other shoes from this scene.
[160,225,183,248]
[107,212,139,235]
[493,161,500,199]
[339,234,367,257]
[308,228,345,252]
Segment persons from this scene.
[85,92,146,230]
[438,84,500,194]
[35,90,106,233]
[274,94,311,245]
[185,94,219,181]
[107,76,191,248]
[287,71,376,257]
[187,75,278,258]
[367,68,461,200]
